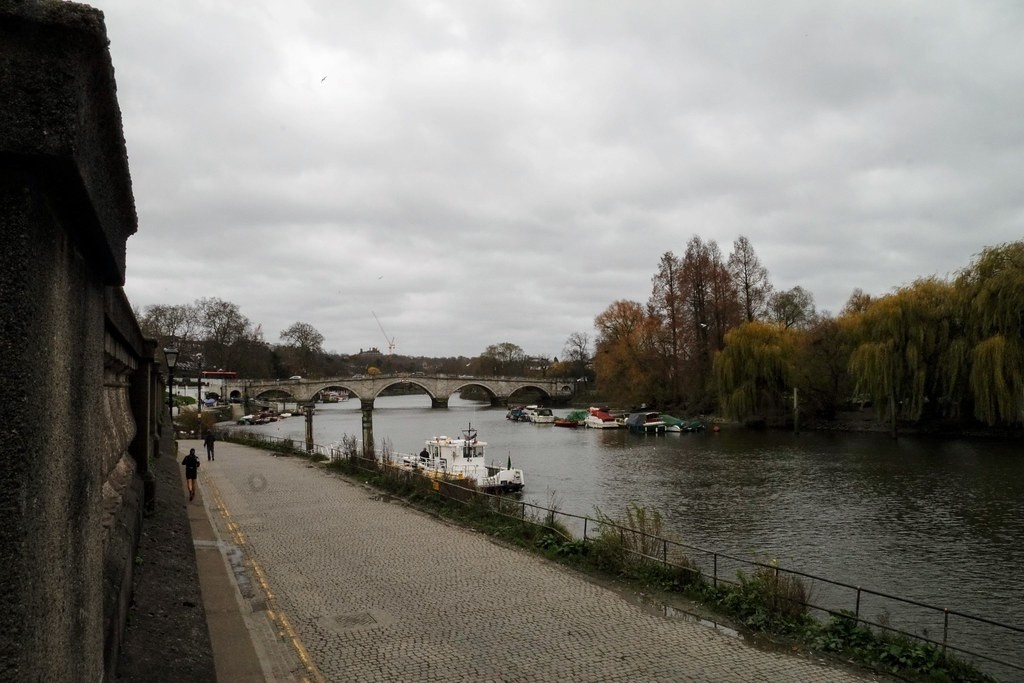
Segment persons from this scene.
[182,448,200,501]
[420,448,430,467]
[204,431,216,461]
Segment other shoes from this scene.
[212,458,214,460]
[189,490,195,501]
[208,457,210,460]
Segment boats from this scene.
[237,410,292,426]
[583,406,619,429]
[553,418,578,427]
[666,423,693,432]
[624,412,666,433]
[505,404,554,424]
[401,421,525,495]
[322,392,338,403]
[338,396,349,402]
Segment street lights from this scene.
[164,345,179,423]
[196,353,203,440]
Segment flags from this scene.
[508,457,511,470]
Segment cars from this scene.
[202,398,216,407]
[577,378,584,382]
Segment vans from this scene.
[288,375,301,380]
[412,372,424,378]
[352,374,362,379]
